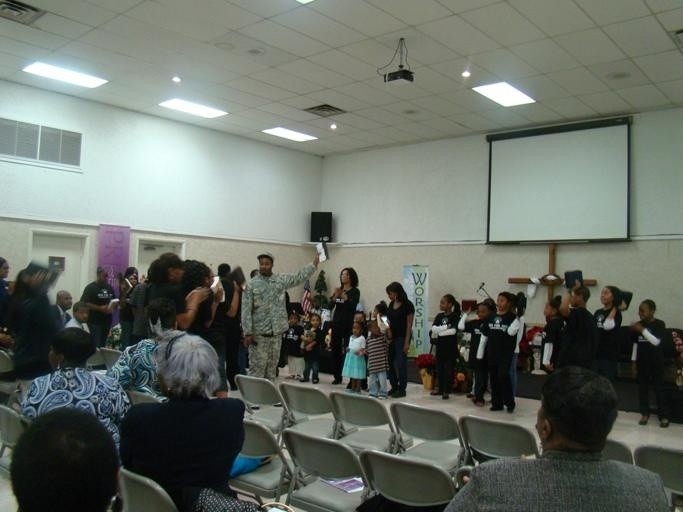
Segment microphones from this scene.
[476,282,485,292]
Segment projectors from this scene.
[384,70,416,97]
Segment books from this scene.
[315,242,329,262]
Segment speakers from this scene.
[311,212,332,242]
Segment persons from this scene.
[0,251,683,512]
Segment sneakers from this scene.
[286,374,409,399]
[639,413,651,425]
[660,417,671,429]
[428,389,517,414]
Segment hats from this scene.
[182,260,210,284]
[256,252,274,260]
